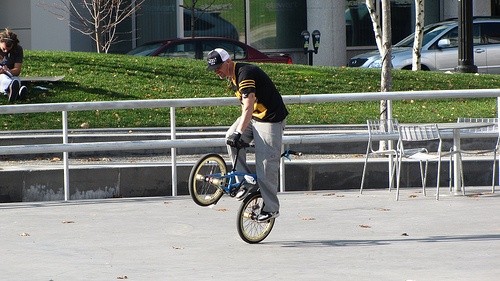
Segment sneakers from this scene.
[236,178,256,202]
[257,211,280,223]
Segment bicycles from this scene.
[187,136,304,244]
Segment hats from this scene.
[205,47,230,72]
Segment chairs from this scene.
[395,123,465,201]
[359,118,429,197]
[448,117,500,194]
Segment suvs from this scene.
[182,9,239,39]
[345,15,500,75]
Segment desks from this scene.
[400,121,494,197]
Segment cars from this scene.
[123,37,293,64]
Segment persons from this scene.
[206,48,289,222]
[0,27,27,105]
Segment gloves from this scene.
[226,131,242,147]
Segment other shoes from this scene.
[18,85,29,100]
[7,79,20,103]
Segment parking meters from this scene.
[301,30,321,65]
